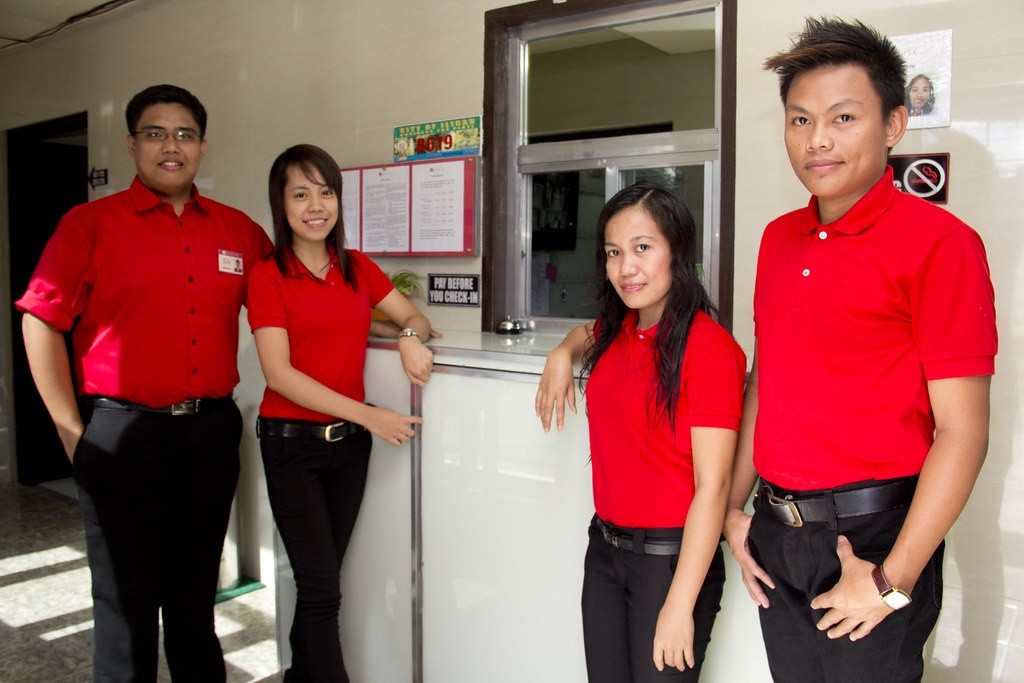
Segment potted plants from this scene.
[373,268,423,321]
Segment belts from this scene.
[595,520,727,554]
[261,415,364,442]
[86,395,232,415]
[759,481,914,528]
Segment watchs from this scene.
[399,328,419,337]
[871,564,912,610]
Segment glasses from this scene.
[129,127,202,142]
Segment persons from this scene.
[14,84,443,683]
[724,13,998,683]
[246,144,434,683]
[903,74,935,117]
[234,260,242,273]
[535,182,747,683]
